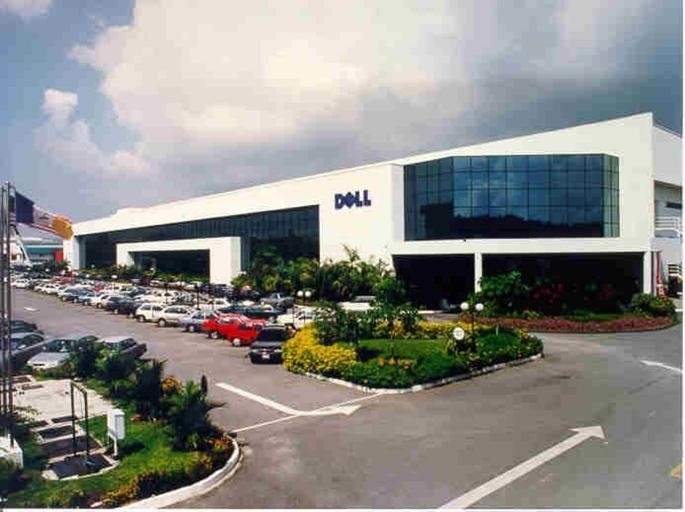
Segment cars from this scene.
[85,336,147,364]
[8,269,336,347]
[249,324,292,364]
[0,319,38,335]
[26,337,84,369]
[0,332,44,370]
[340,296,383,317]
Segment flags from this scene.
[5,188,74,268]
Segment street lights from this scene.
[460,302,484,353]
